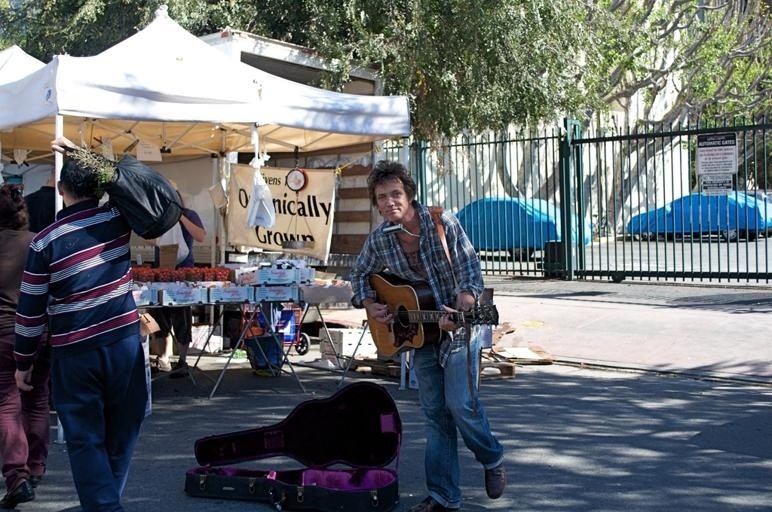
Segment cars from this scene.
[625,190,771,242]
[450,197,590,261]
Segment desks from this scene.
[134,299,369,400]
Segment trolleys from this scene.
[237,297,310,355]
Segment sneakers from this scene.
[170,361,189,379]
[150,359,172,373]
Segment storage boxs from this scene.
[135,252,354,302]
[320,329,378,361]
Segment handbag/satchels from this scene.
[245,333,283,376]
[66,147,182,240]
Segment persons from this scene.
[0,184,50,510]
[351,156,506,512]
[142,187,206,378]
[12,157,147,511]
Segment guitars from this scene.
[366,270,499,359]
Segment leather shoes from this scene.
[29,476,42,488]
[0,481,35,509]
[485,463,506,499]
[408,495,457,512]
